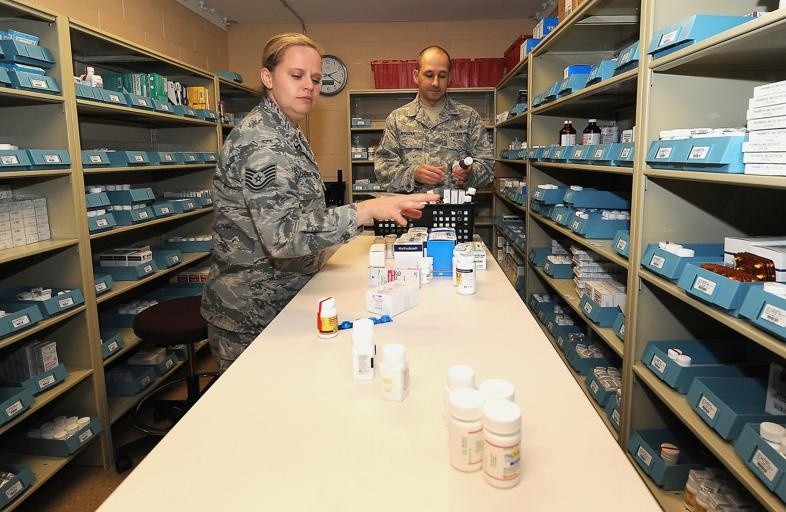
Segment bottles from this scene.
[677,355,692,366]
[418,258,435,283]
[316,295,338,339]
[168,233,213,241]
[352,318,375,378]
[452,242,477,296]
[657,127,748,139]
[760,420,785,449]
[378,342,410,401]
[657,239,695,258]
[547,254,573,264]
[593,366,623,390]
[24,415,90,440]
[560,120,579,147]
[352,136,362,153]
[0,143,19,152]
[73,67,105,88]
[533,292,574,326]
[446,365,522,489]
[118,297,158,315]
[539,183,630,222]
[583,120,601,146]
[667,348,682,360]
[574,342,606,358]
[685,469,747,511]
[521,142,527,149]
[85,183,147,216]
[661,442,683,467]
[599,125,618,143]
[451,156,473,174]
[367,147,375,161]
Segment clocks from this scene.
[315,53,349,97]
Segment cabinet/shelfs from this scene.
[213,74,260,155]
[62,12,223,466]
[0,0,115,510]
[487,48,531,306]
[617,1,786,512]
[344,86,496,254]
[524,0,648,446]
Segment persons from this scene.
[374,47,495,190]
[198,32,439,381]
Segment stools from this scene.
[129,293,224,440]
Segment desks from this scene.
[93,222,667,511]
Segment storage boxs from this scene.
[364,223,489,317]
[765,362,785,422]
[368,32,532,91]
[166,266,214,287]
[492,231,525,285]
[581,276,626,312]
[97,242,153,270]
[100,68,211,114]
[517,0,582,65]
